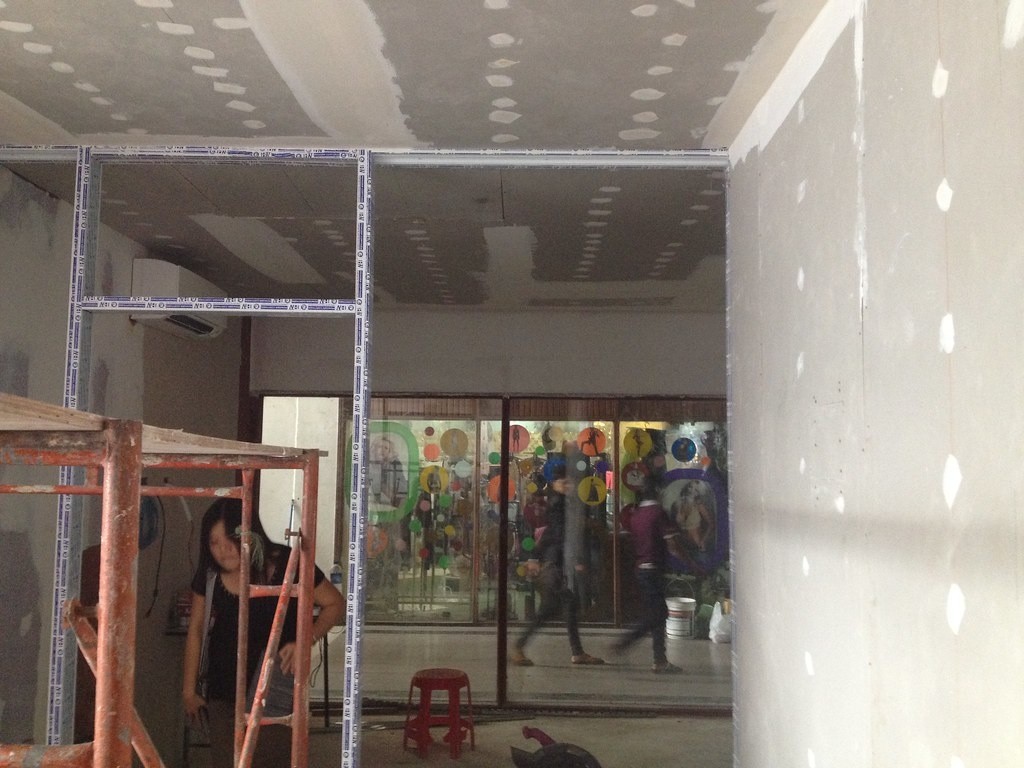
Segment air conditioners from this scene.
[130,258,228,345]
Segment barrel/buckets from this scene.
[666,579,697,640]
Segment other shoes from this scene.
[572,654,605,665]
[507,650,534,668]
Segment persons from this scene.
[609,473,708,674]
[512,465,605,667]
[181,496,347,768]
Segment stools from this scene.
[404,668,475,759]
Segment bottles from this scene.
[330,561,342,595]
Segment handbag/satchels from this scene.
[708,600,733,644]
[182,678,211,746]
[535,569,579,609]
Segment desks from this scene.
[162,615,328,768]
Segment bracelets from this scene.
[313,635,316,644]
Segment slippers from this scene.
[651,661,683,675]
